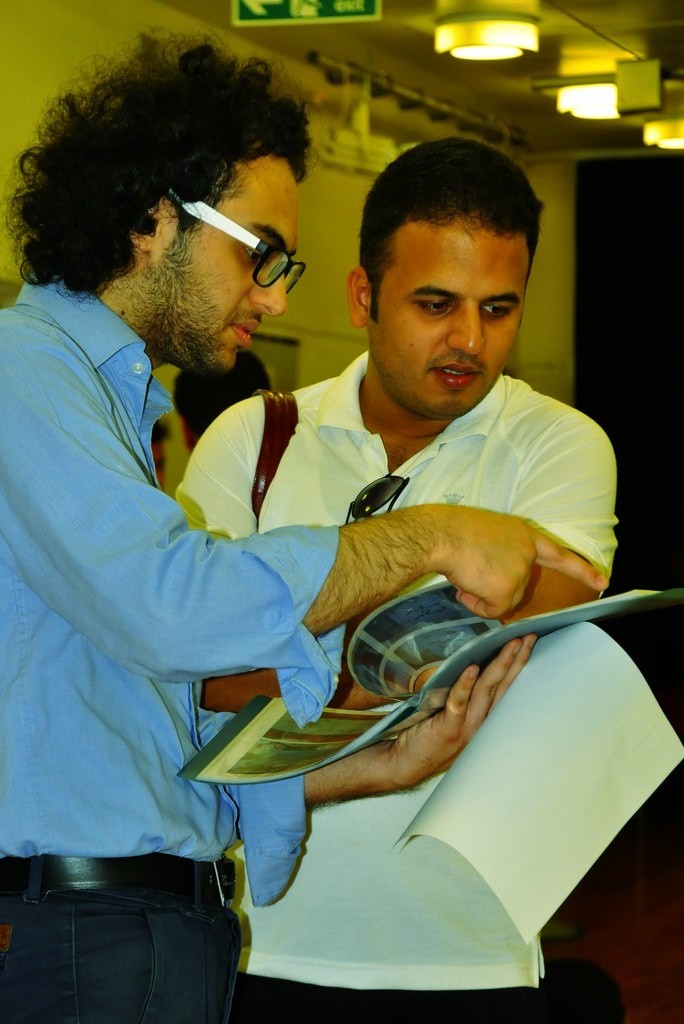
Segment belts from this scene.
[1,855,236,907]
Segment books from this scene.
[176,579,684,786]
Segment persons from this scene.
[0,27,619,1024]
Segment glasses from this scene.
[345,473,410,525]
[167,188,307,295]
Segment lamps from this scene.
[555,36,648,121]
[640,79,684,150]
[430,0,541,61]
